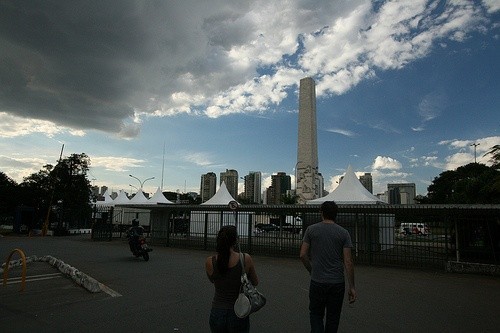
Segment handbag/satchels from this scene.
[234,251,266,317]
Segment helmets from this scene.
[132,218,139,224]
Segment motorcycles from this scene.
[127,229,153,262]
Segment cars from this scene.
[260,223,279,231]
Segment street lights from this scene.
[129,175,155,189]
[129,184,138,190]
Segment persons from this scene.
[129,217,145,257]
[205,225,259,333]
[299,200,356,332]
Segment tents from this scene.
[91,185,174,229]
[304,163,396,249]
[190,180,256,239]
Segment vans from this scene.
[399,222,432,236]
